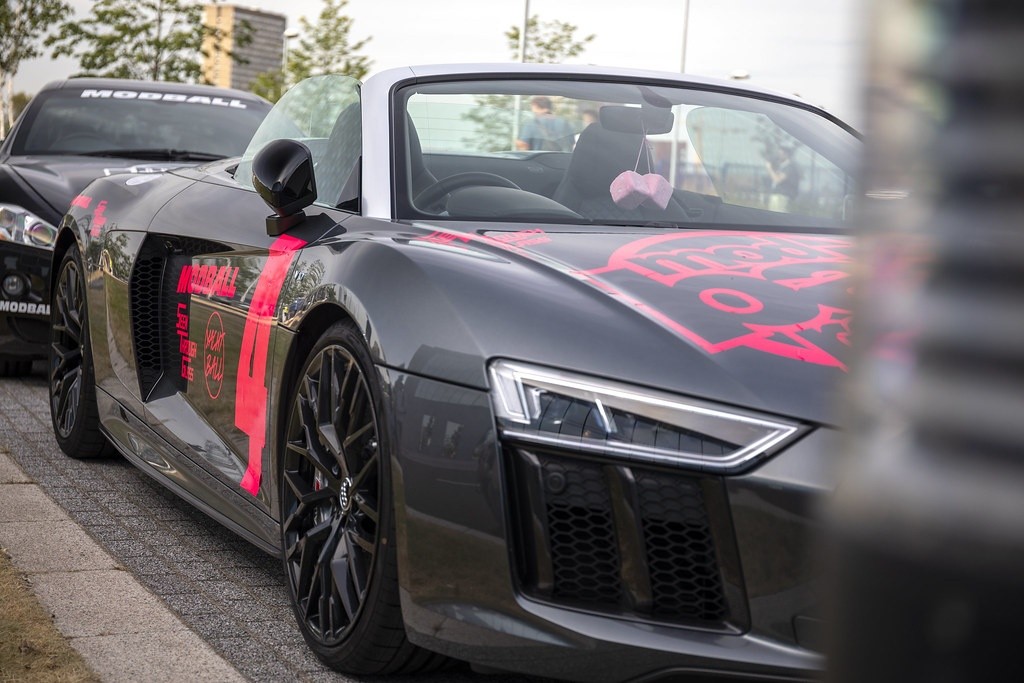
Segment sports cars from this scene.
[47,65,922,683]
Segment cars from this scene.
[2,77,274,379]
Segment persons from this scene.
[763,146,797,213]
[516,95,574,153]
[573,108,600,149]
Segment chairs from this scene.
[552,122,660,222]
[314,102,452,218]
